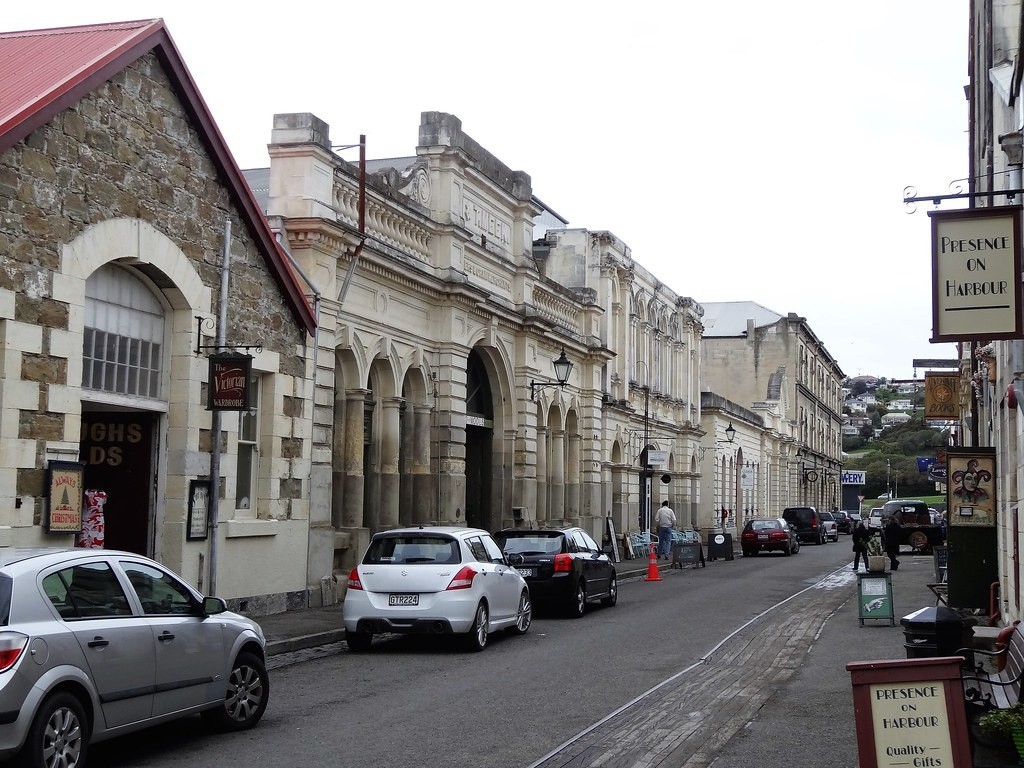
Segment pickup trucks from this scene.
[867,508,884,535]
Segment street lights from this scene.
[887,459,890,501]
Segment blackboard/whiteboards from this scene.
[932,546,949,583]
[673,542,705,562]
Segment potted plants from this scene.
[862,535,886,571]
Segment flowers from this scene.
[975,346,994,369]
[969,374,983,398]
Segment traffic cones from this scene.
[644,543,663,581]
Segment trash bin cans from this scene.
[900,605,980,671]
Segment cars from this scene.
[741,518,800,556]
[819,511,866,542]
[342,526,533,652]
[878,493,891,500]
[0,546,269,768]
[494,527,617,618]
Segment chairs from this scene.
[630,530,699,559]
[58,577,115,620]
[518,540,559,552]
[927,562,948,607]
[399,543,421,559]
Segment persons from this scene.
[834,507,839,512]
[884,515,902,570]
[852,522,870,572]
[79,469,106,549]
[943,510,947,519]
[655,500,676,560]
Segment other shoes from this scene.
[657,555,661,559]
[853,568,858,570]
[895,561,899,569]
[665,556,669,560]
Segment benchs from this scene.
[946,621,1024,768]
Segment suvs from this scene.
[782,506,828,545]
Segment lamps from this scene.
[530,347,573,401]
[820,464,833,477]
[787,450,802,469]
[717,421,736,443]
